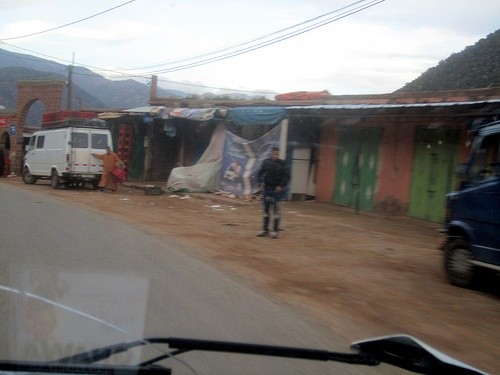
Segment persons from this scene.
[257,146,290,238]
[90,146,125,193]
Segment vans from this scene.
[436,119,500,286]
[21,126,114,189]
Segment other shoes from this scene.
[258,231,268,237]
[271,231,278,239]
